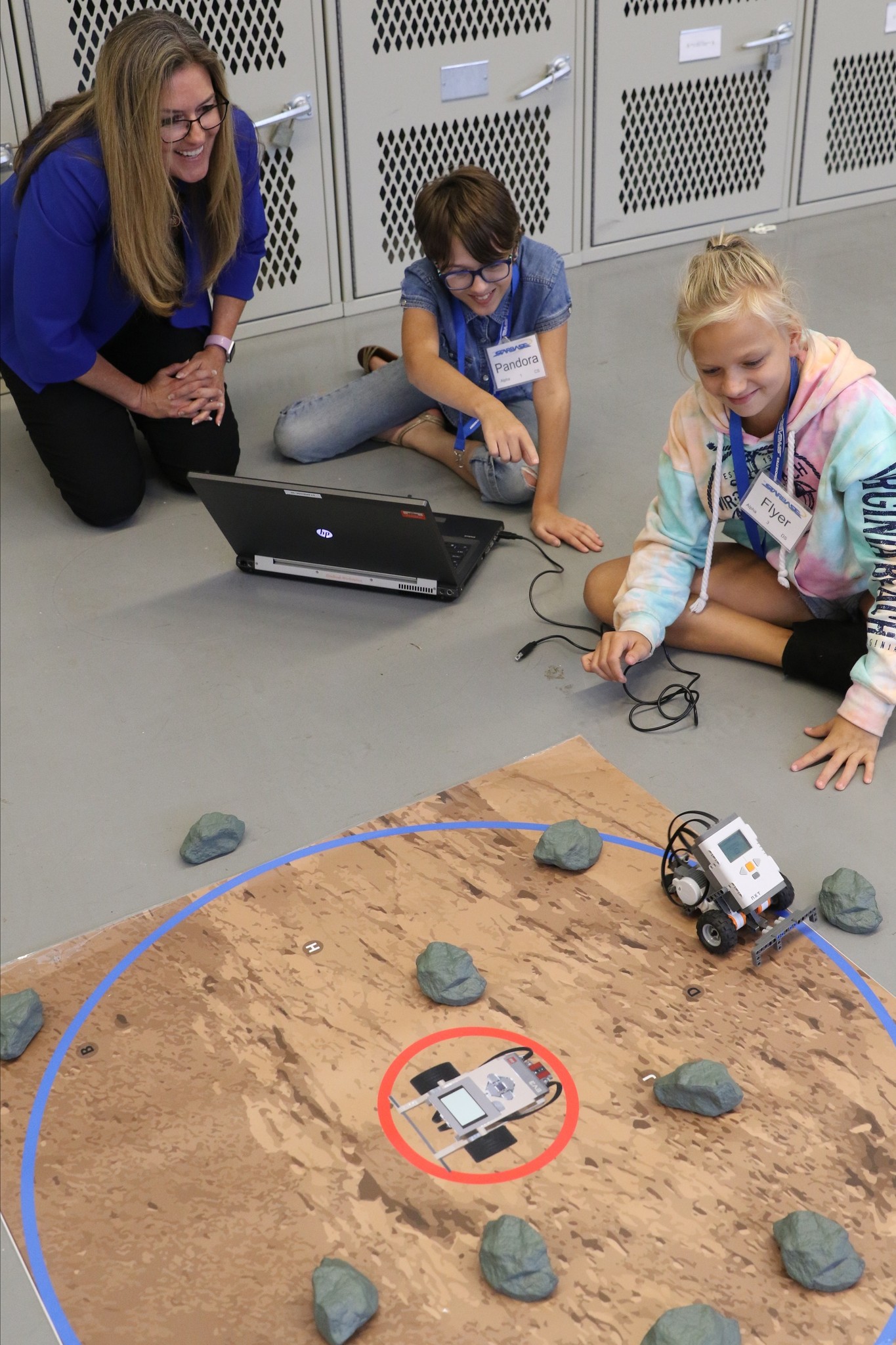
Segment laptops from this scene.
[187,473,506,604]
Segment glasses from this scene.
[161,94,230,143]
[433,254,512,290]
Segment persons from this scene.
[273,166,606,554]
[0,10,269,528]
[581,236,896,791]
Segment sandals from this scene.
[358,345,400,373]
[376,412,444,446]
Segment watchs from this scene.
[204,335,235,363]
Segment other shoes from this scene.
[781,617,868,697]
